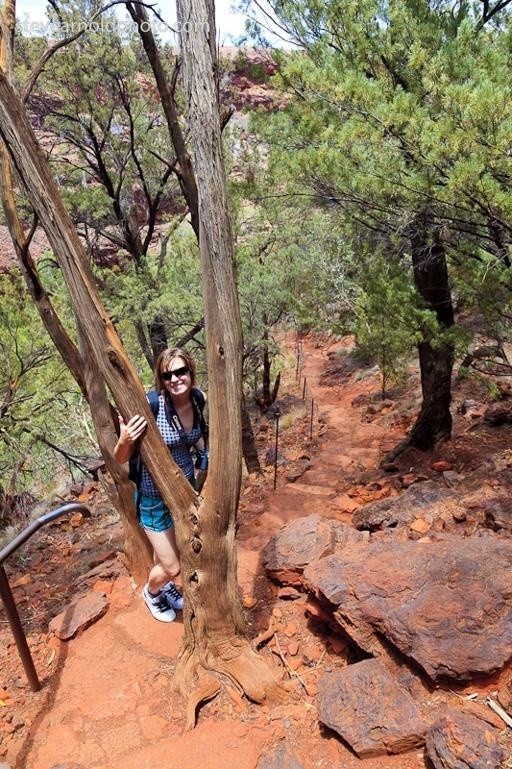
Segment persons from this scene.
[111,345,213,626]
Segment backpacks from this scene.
[129,389,205,491]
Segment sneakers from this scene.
[160,581,184,611]
[142,584,176,623]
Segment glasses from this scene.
[161,366,189,381]
